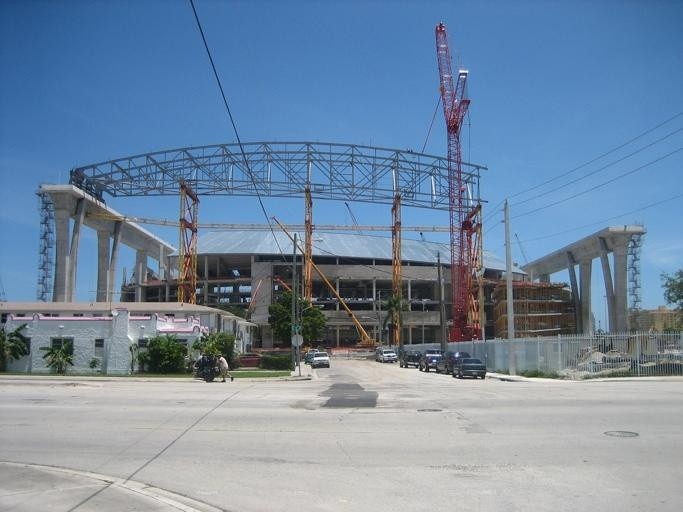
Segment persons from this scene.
[217,354,233,382]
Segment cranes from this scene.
[433,21,484,342]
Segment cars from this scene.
[304,348,331,369]
[233,353,265,369]
[374,348,486,380]
